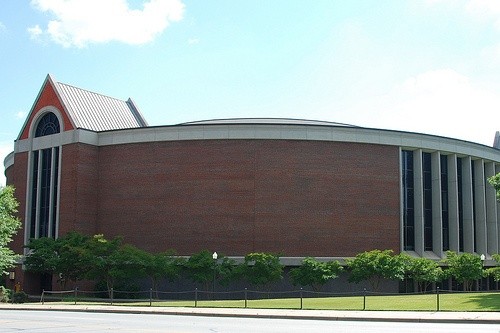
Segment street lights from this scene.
[8,268,16,292]
[213,251,218,298]
[480,254,485,291]
